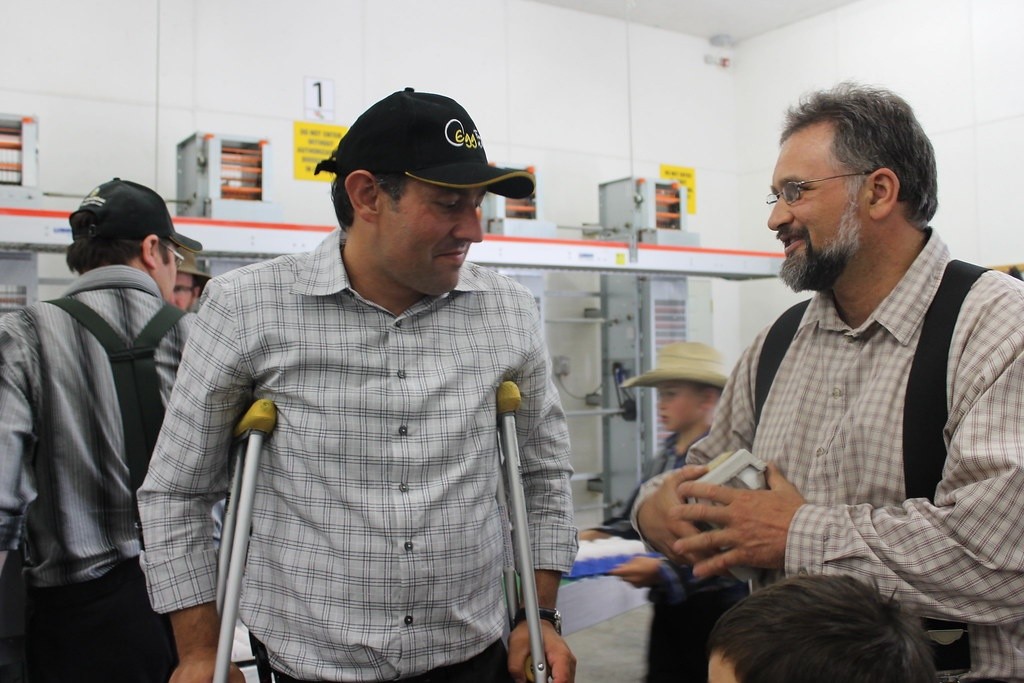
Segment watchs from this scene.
[510,608,562,635]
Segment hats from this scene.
[67,178,203,255]
[618,342,727,390]
[314,88,535,201]
[178,249,211,282]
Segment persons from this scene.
[135,88,579,683]
[576,342,750,683]
[708,573,939,683]
[1,177,239,683]
[631,83,1022,683]
[162,233,212,313]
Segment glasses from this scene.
[765,170,869,206]
